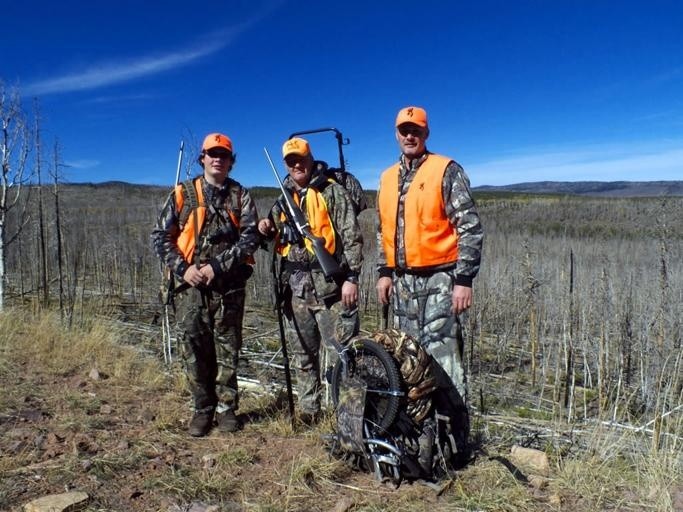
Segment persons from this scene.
[256,136,365,432]
[150,132,260,437]
[375,106,484,407]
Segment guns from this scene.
[160,141,185,304]
[263,147,343,276]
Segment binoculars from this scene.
[279,223,297,244]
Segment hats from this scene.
[202,133,232,153]
[282,138,310,160]
[395,106,427,129]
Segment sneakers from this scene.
[216,410,239,432]
[189,407,214,436]
